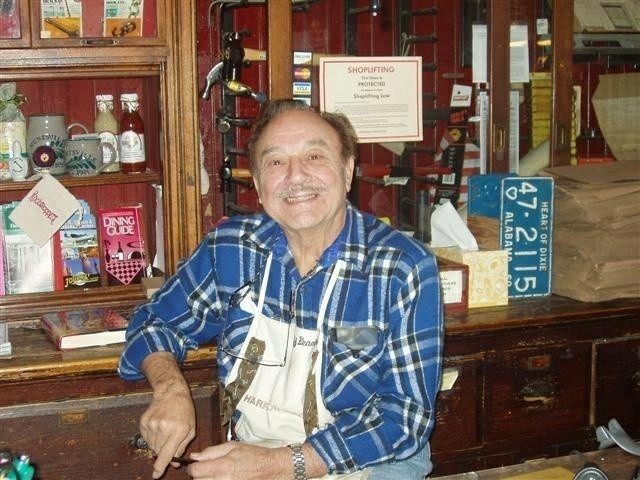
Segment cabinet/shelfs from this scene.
[0,0,203,322]
[428,294,640,474]
[0,321,234,480]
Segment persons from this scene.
[114,99,445,480]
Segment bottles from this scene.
[8,139,29,183]
[94,93,133,174]
[119,92,147,175]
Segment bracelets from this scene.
[288,443,306,480]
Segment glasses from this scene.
[217,280,293,368]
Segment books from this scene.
[40,305,129,351]
[98,205,151,286]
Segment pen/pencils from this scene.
[152,455,199,466]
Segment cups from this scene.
[62,137,117,178]
[25,110,89,177]
